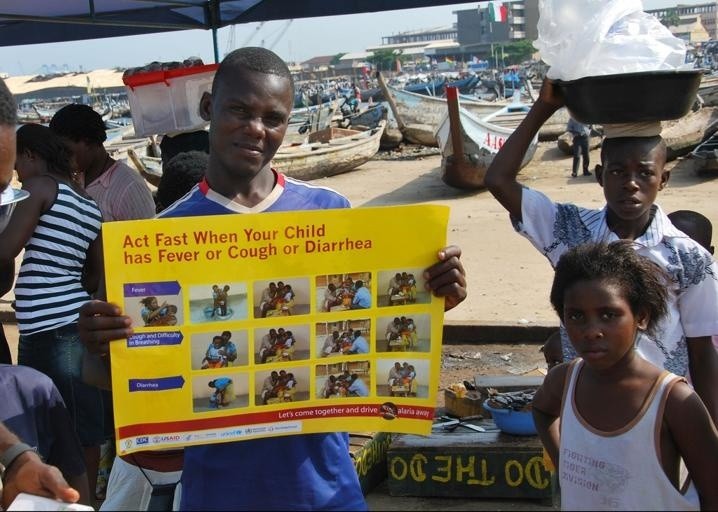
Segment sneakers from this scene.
[571,172,577,177]
[583,172,592,176]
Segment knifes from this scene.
[432,413,483,428]
[440,416,486,433]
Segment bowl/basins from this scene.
[552,66,711,122]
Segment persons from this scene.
[483,78,718,429]
[531,241,717,511]
[566,117,602,177]
[666,210,715,255]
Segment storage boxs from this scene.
[122,63,220,138]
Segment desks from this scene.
[391,384,410,398]
[389,339,408,352]
[391,294,408,307]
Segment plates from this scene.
[5,492,94,512]
[0,187,31,207]
